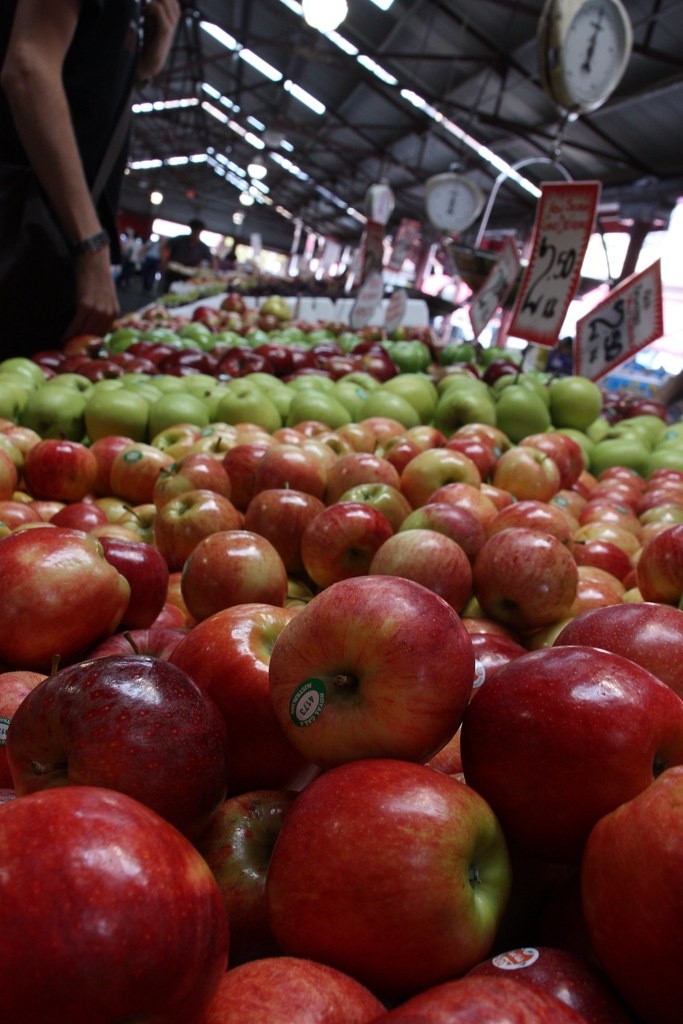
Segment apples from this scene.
[0,278,683,1024]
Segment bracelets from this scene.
[70,230,110,255]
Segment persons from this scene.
[111,219,236,297]
[0,0,185,362]
